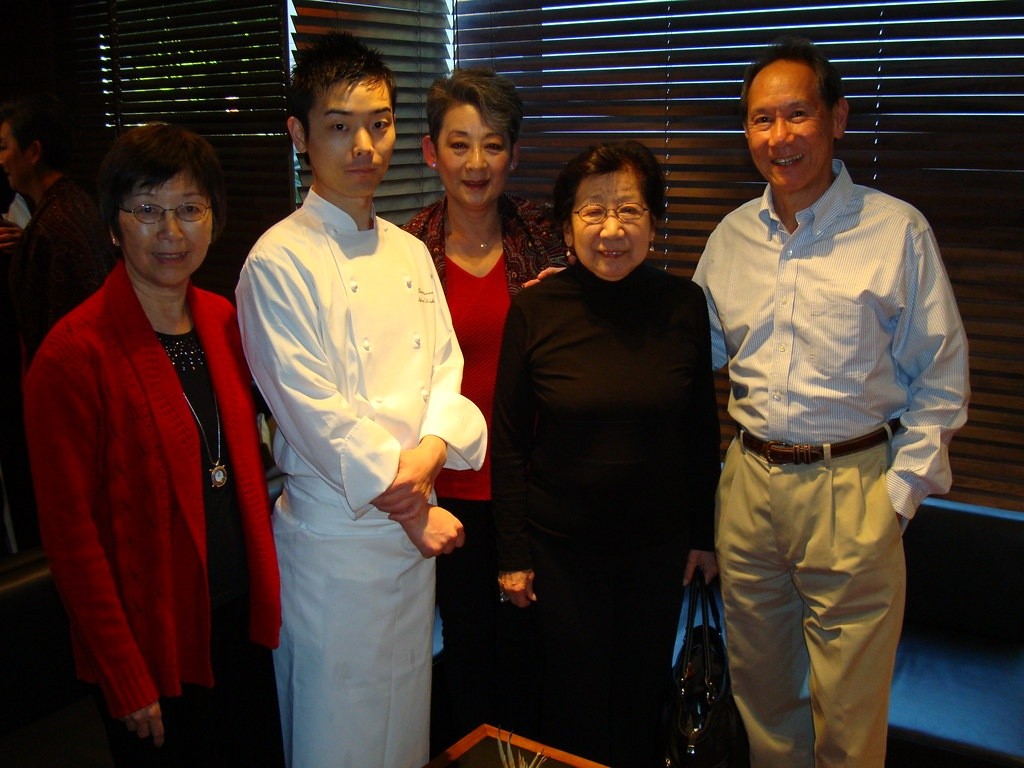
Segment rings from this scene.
[500,592,510,601]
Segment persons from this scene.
[491,140,720,768]
[0,100,116,563]
[25,126,281,768]
[235,30,487,768]
[691,40,971,768]
[402,72,570,763]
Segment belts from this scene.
[736,417,900,465]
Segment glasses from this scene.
[119,201,214,225]
[573,202,650,225]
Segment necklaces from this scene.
[446,218,500,252]
[182,391,228,487]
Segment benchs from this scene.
[668,461,1024,768]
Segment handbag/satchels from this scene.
[660,564,751,768]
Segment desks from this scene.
[421,723,613,768]
